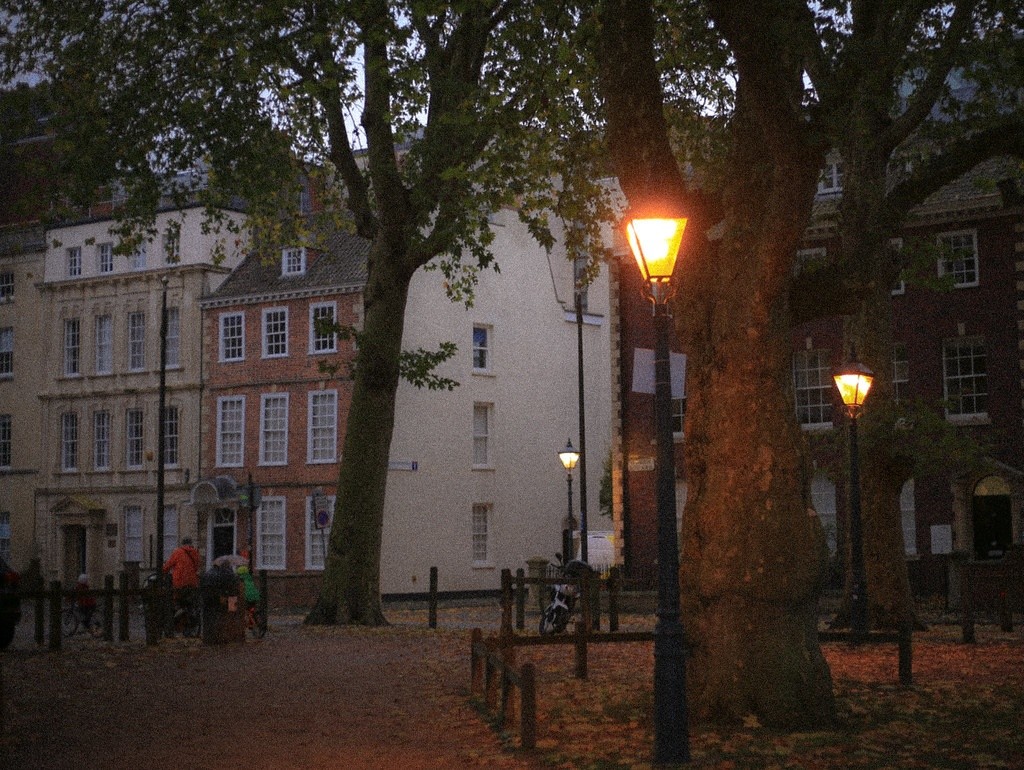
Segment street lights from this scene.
[622,175,694,768]
[560,437,583,558]
[831,341,875,634]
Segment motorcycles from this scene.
[538,552,600,637]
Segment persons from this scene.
[236,566,259,609]
[0,558,28,650]
[71,575,97,633]
[163,536,203,619]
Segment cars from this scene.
[0,556,21,647]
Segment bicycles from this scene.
[245,603,267,640]
[60,596,105,638]
[142,573,202,640]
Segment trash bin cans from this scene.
[198,572,246,642]
